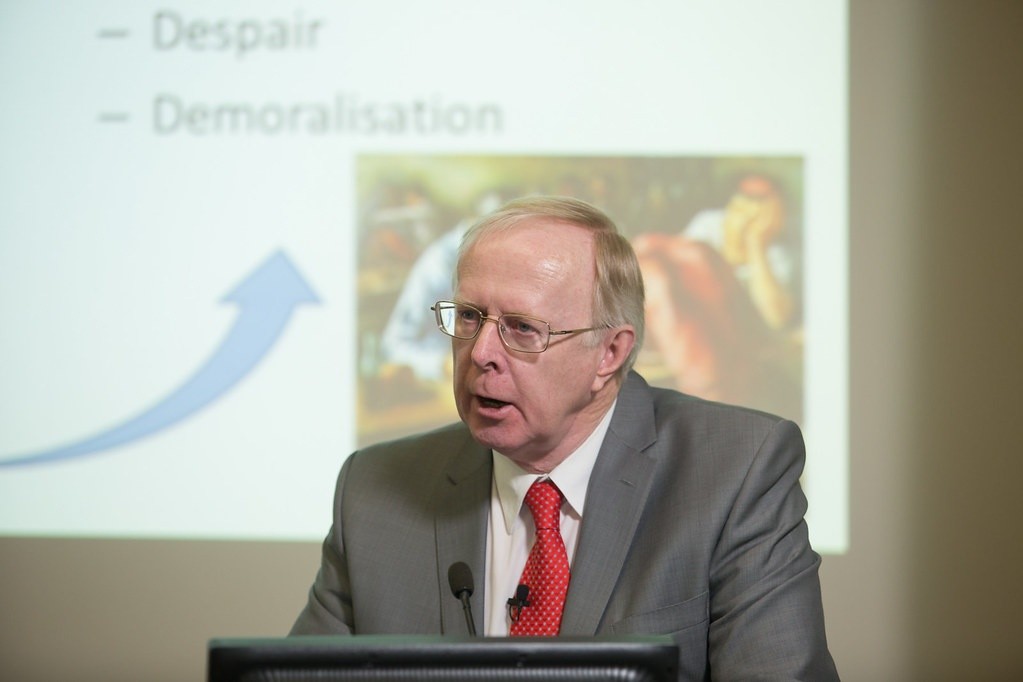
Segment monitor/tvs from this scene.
[206,633,679,682]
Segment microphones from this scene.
[448,561,477,637]
[506,584,530,621]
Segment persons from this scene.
[691,179,795,326]
[286,195,838,682]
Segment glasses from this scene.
[431,300,614,353]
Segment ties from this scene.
[509,482,570,637]
